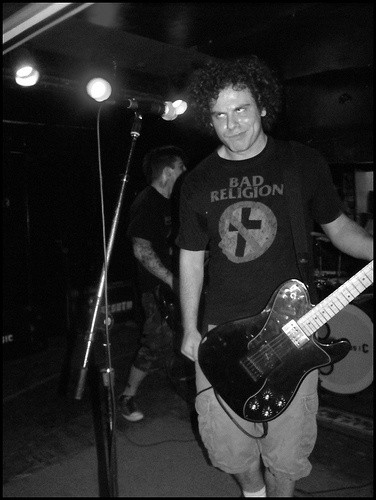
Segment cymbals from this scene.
[310,232,330,244]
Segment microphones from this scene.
[108,99,178,121]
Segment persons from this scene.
[116,148,192,420]
[170,60,373,498]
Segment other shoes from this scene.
[116,393,145,421]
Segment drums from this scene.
[344,211,368,228]
[352,294,376,315]
[315,277,350,300]
[317,303,374,394]
[312,269,347,277]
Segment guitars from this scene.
[157,275,206,363]
[199,262,375,422]
